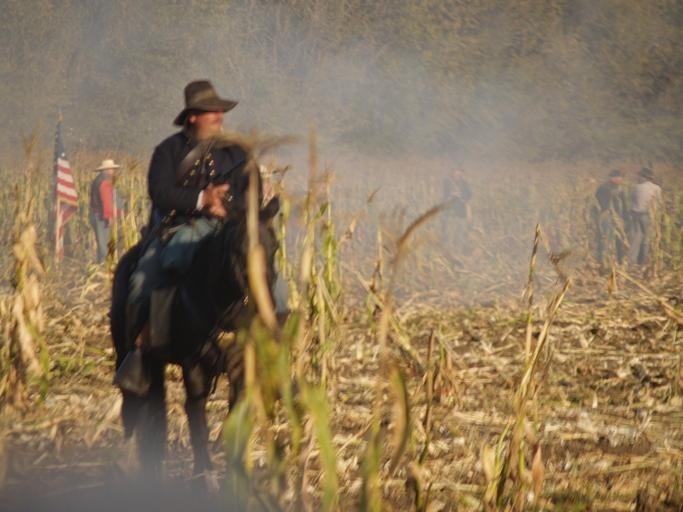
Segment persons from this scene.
[626,164,662,266]
[434,163,472,252]
[595,168,629,269]
[87,157,125,268]
[102,73,291,394]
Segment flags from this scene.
[46,121,79,250]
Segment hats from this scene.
[95,159,120,172]
[173,80,238,125]
[639,167,656,178]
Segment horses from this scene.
[108,192,281,495]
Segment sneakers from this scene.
[114,345,151,397]
